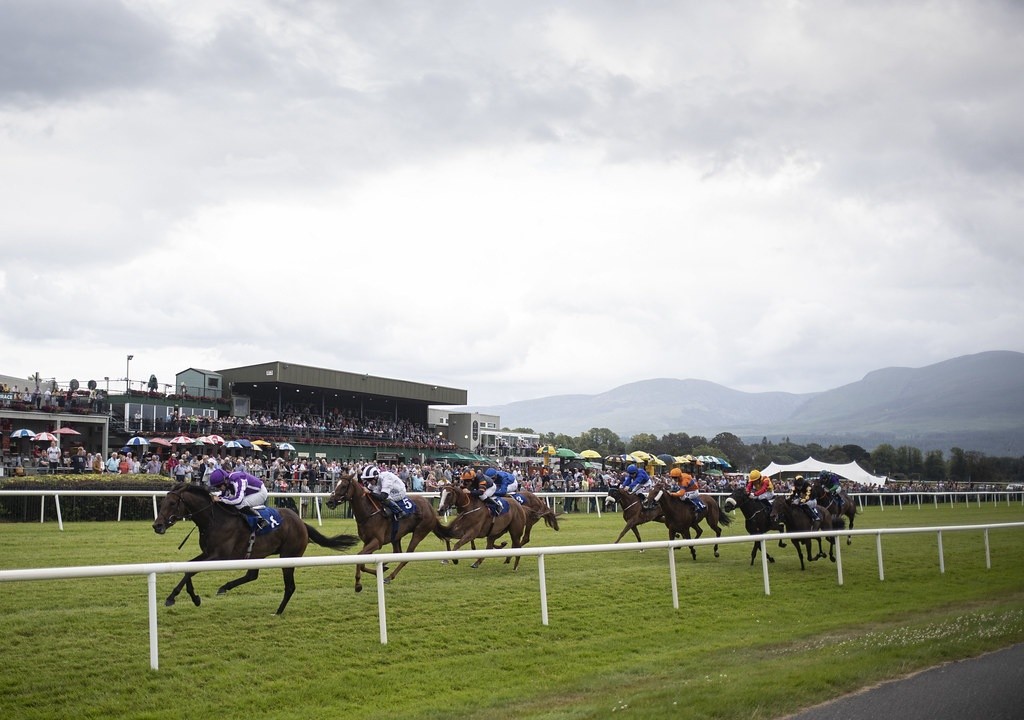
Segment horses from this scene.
[505,491,559,547]
[770,496,835,568]
[437,483,534,571]
[646,481,733,560]
[809,481,857,543]
[152,482,359,616]
[605,483,667,543]
[724,487,787,564]
[326,472,458,592]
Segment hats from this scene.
[171,453,177,458]
[515,467,623,477]
[698,476,738,486]
[364,460,450,468]
[278,458,284,462]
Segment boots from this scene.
[385,499,407,519]
[685,498,699,513]
[638,493,654,507]
[834,494,845,506]
[482,497,502,517]
[760,499,773,514]
[240,506,270,533]
[801,504,819,524]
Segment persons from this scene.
[565,467,974,521]
[0,382,104,415]
[0,419,565,541]
[133,401,456,445]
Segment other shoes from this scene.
[564,511,568,514]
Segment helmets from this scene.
[484,468,497,477]
[749,470,761,481]
[627,464,638,474]
[361,466,379,478]
[210,468,229,486]
[794,475,805,486]
[460,469,476,480]
[668,468,682,477]
[820,470,829,479]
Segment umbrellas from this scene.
[126,434,295,457]
[536,444,732,474]
[50,427,80,434]
[29,432,57,441]
[9,429,36,438]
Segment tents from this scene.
[759,455,888,488]
